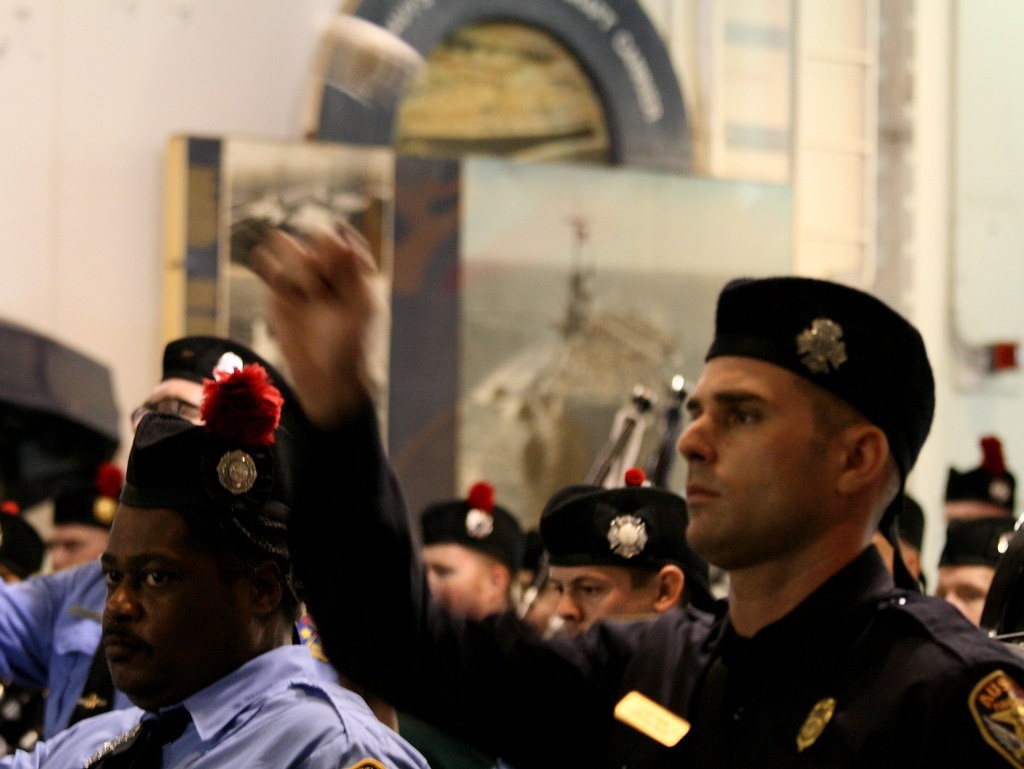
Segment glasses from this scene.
[128,393,201,432]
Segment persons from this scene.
[250,222,1024,769]
[0,334,1024,769]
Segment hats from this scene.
[538,466,709,602]
[881,493,926,550]
[114,364,293,575]
[52,461,125,530]
[702,275,938,491]
[418,479,528,575]
[944,435,1017,511]
[0,499,46,573]
[938,515,1023,569]
[158,334,295,431]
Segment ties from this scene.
[67,633,114,730]
[85,707,191,769]
